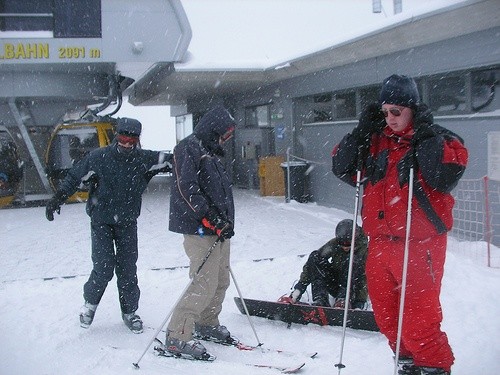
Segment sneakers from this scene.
[122,310,144,334]
[165,333,206,357]
[192,322,231,343]
[311,296,351,309]
[78,298,99,329]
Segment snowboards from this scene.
[234,297,380,333]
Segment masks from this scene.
[117,144,135,158]
[220,133,234,140]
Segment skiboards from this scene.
[155,328,318,374]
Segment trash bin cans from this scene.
[279,161,311,203]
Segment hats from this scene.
[116,117,142,136]
[335,219,358,240]
[378,73,421,109]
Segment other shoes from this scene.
[392,356,451,375]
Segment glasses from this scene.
[338,236,353,247]
[116,135,139,144]
[377,106,407,118]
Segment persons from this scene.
[165,108,236,355]
[46,117,173,333]
[332,73,468,375]
[287,219,369,311]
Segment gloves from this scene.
[351,102,387,142]
[287,288,302,300]
[45,191,68,222]
[352,300,368,310]
[196,208,234,242]
[412,102,437,141]
